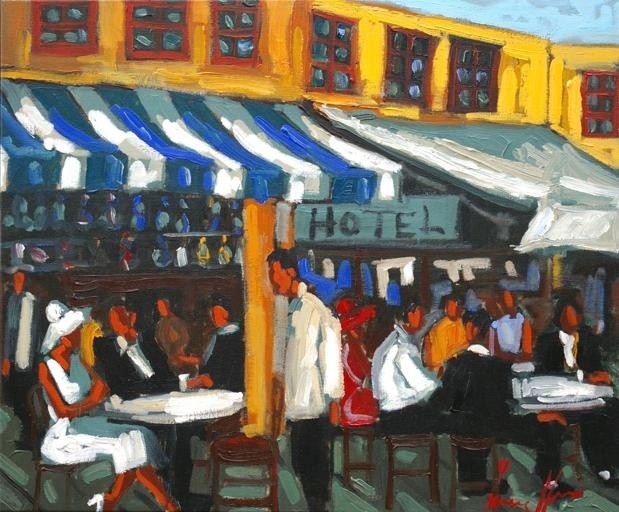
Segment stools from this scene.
[341,423,502,511]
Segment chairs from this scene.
[24,384,91,512]
[210,433,279,511]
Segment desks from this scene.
[511,372,605,489]
[105,388,248,512]
